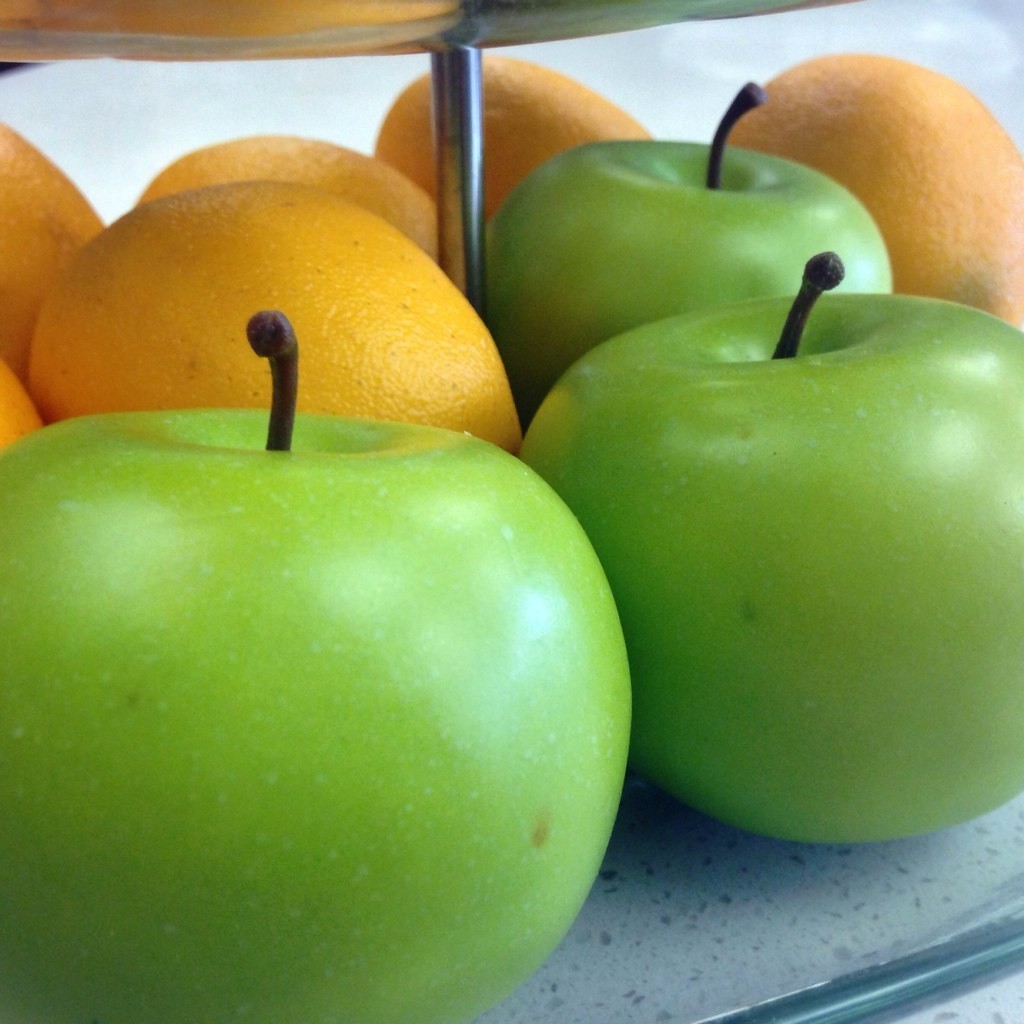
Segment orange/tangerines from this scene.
[0,37,1024,458]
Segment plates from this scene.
[1,0,1024,1024]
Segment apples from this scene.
[1,306,634,1024]
[510,253,1024,841]
[483,78,891,438]
